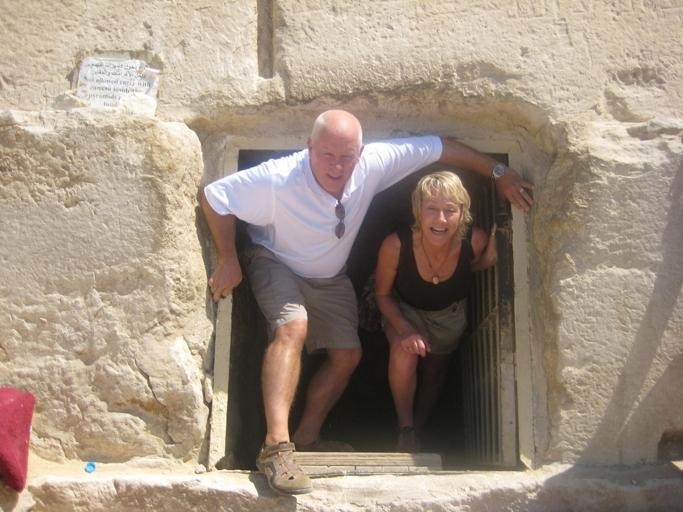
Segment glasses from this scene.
[335,199,345,241]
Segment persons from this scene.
[201,106,535,496]
[374,169,498,451]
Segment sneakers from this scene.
[394,425,425,453]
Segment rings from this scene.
[519,188,522,194]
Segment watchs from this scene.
[489,163,506,181]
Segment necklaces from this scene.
[421,232,453,286]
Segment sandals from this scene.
[255,440,313,495]
[292,438,354,452]
